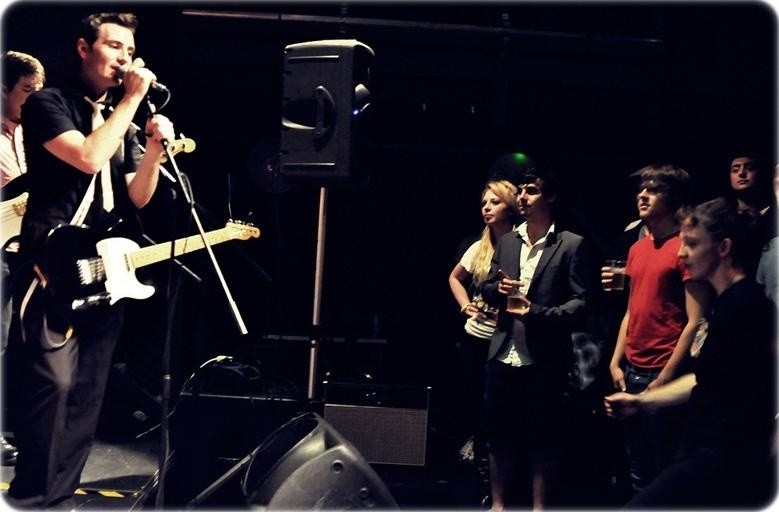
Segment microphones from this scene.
[119,65,170,98]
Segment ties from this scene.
[87,98,117,215]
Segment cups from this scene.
[507,293,522,310]
[604,260,627,291]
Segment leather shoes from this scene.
[0,437,20,466]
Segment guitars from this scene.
[43,220,262,311]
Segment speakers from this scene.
[241,412,401,511]
[277,37,375,180]
[322,403,430,470]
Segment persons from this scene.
[448,153,778,510]
[7,11,177,509]
[0,49,47,469]
